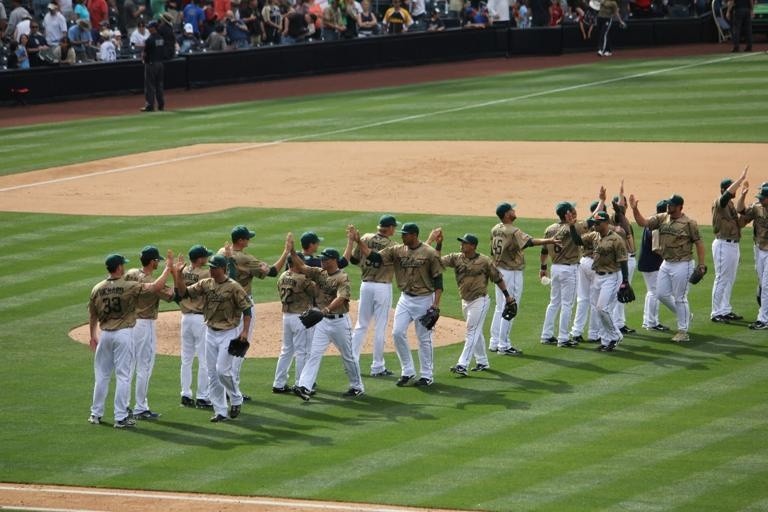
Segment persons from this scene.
[270,214,446,402]
[139,21,165,111]
[0,1,756,70]
[90,225,292,428]
[708,166,768,331]
[536,180,705,348]
[434,203,562,376]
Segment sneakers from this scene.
[748,319,767,331]
[341,388,363,398]
[623,323,635,335]
[211,407,242,424]
[710,311,744,325]
[86,415,102,425]
[448,362,469,376]
[471,364,491,373]
[372,369,395,379]
[398,375,434,387]
[272,380,318,402]
[497,343,524,359]
[540,332,622,350]
[113,407,159,429]
[649,320,672,332]
[179,396,213,409]
[670,331,692,343]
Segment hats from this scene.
[590,200,610,226]
[139,245,165,261]
[188,245,228,267]
[377,214,403,227]
[231,224,256,239]
[75,18,90,31]
[158,11,174,27]
[656,194,684,212]
[612,195,629,209]
[719,178,737,192]
[105,253,129,269]
[555,200,577,215]
[398,223,421,234]
[317,248,339,260]
[493,202,517,216]
[183,22,194,34]
[299,229,324,246]
[755,183,768,199]
[456,232,480,247]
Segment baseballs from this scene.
[541,276,550,285]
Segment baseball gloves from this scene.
[617,284,635,302]
[502,298,517,321]
[227,337,251,358]
[419,306,440,330]
[298,309,324,329]
[690,267,709,284]
